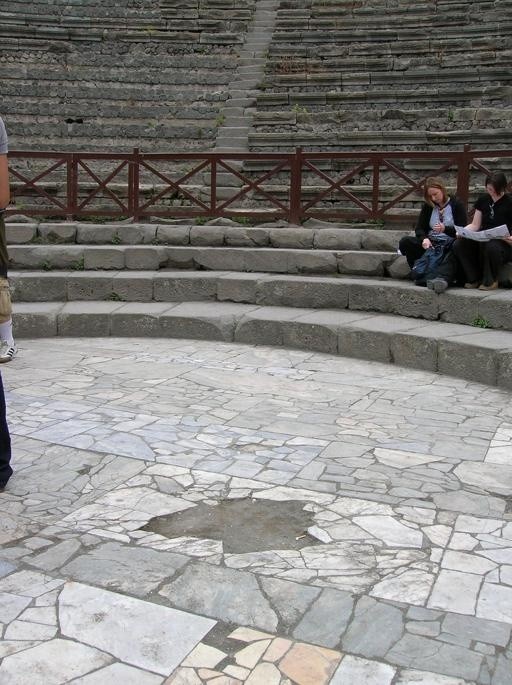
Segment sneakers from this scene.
[0,340,18,363]
[464,279,499,291]
[427,277,448,294]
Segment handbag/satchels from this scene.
[411,245,445,280]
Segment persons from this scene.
[0,116,18,493]
[398,170,512,294]
